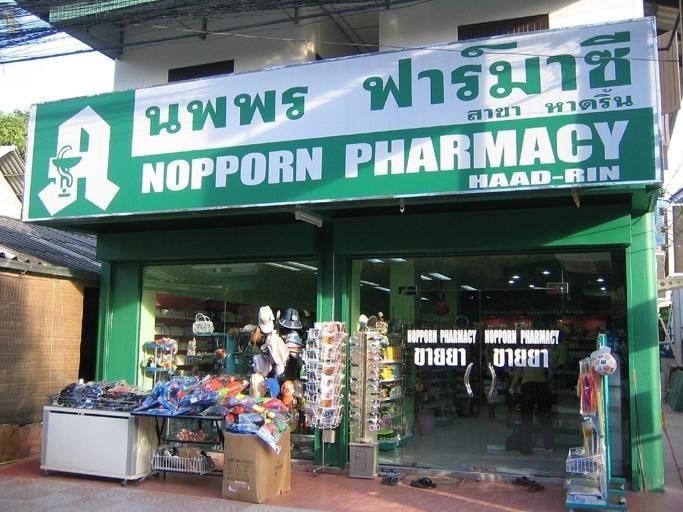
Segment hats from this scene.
[249,306,304,405]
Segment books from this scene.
[303,321,348,430]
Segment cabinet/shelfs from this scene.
[419,368,480,425]
[155,332,251,370]
[143,343,173,372]
[377,360,412,450]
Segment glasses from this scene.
[349,337,382,431]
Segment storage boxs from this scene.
[222,429,292,503]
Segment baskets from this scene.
[151,453,212,475]
[566,447,600,474]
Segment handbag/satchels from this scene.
[193,313,214,334]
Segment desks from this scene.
[131,411,225,481]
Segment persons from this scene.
[549,329,567,380]
[506,364,555,450]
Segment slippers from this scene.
[411,478,435,488]
[380,468,400,486]
[512,476,544,492]
